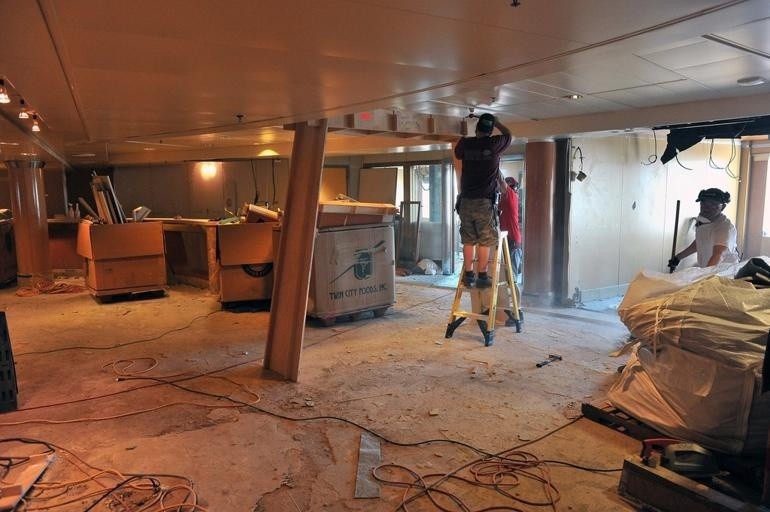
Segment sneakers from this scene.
[505,311,524,326]
[476,276,493,288]
[461,274,475,286]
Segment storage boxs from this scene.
[206,220,396,315]
[74,216,169,289]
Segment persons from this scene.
[452,111,513,289]
[497,170,525,327]
[666,188,741,274]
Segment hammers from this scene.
[536,353,562,368]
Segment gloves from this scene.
[668,258,679,271]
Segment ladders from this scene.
[445,231,522,347]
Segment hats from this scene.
[696,188,728,204]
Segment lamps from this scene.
[0,74,42,132]
